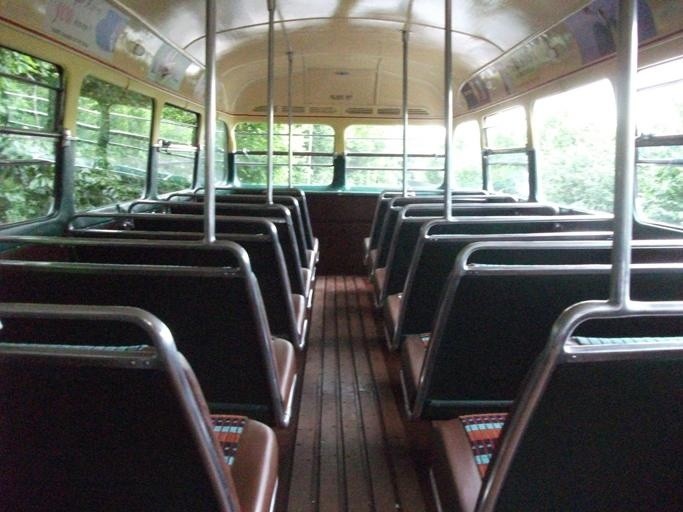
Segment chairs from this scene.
[0,188,683,511]
